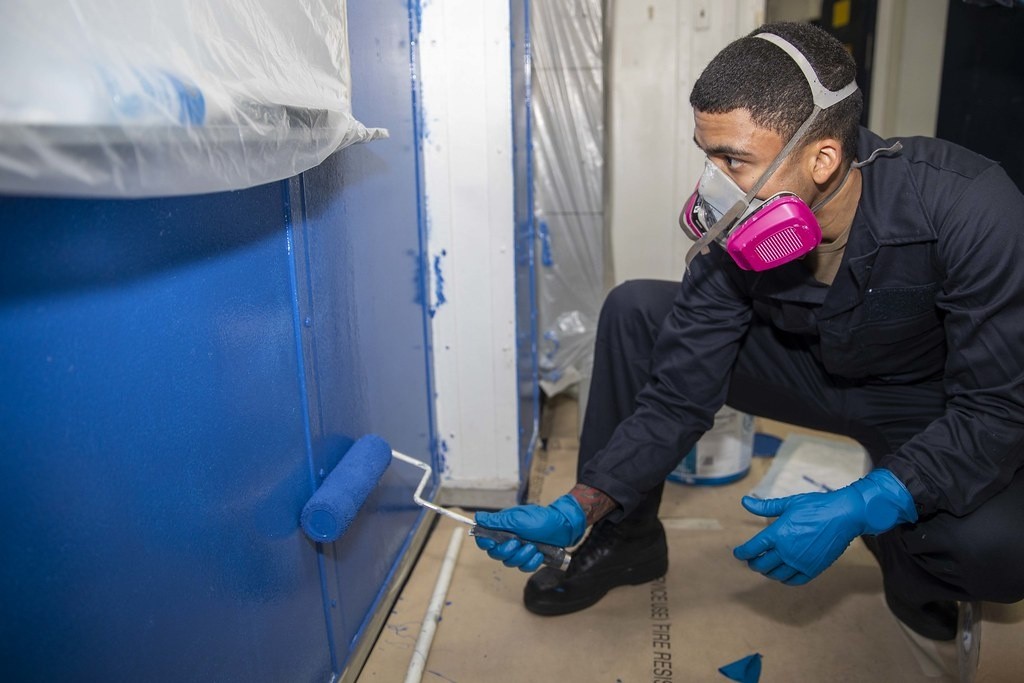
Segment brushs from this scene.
[298,432,573,572]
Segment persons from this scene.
[476,22,1023,643]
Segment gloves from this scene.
[471,495,590,572]
[733,468,920,586]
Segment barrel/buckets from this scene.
[667,403,755,488]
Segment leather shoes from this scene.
[863,530,958,642]
[525,520,670,618]
